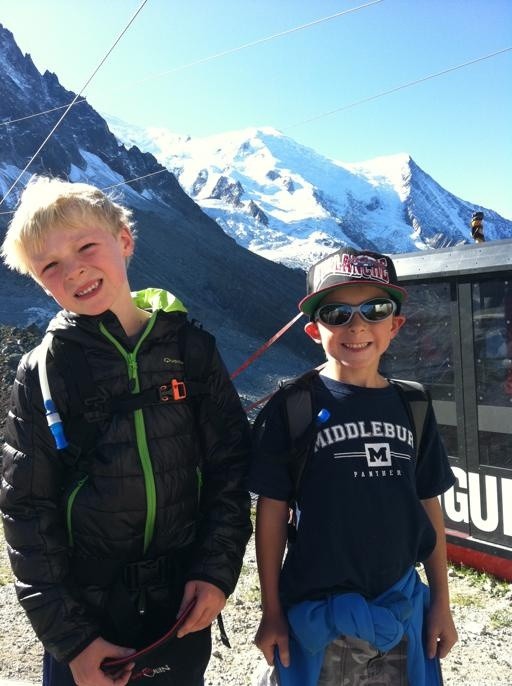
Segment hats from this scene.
[102,598,213,685]
[297,248,407,317]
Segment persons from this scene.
[239,246,458,686]
[1,174,249,686]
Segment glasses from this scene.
[312,297,399,327]
